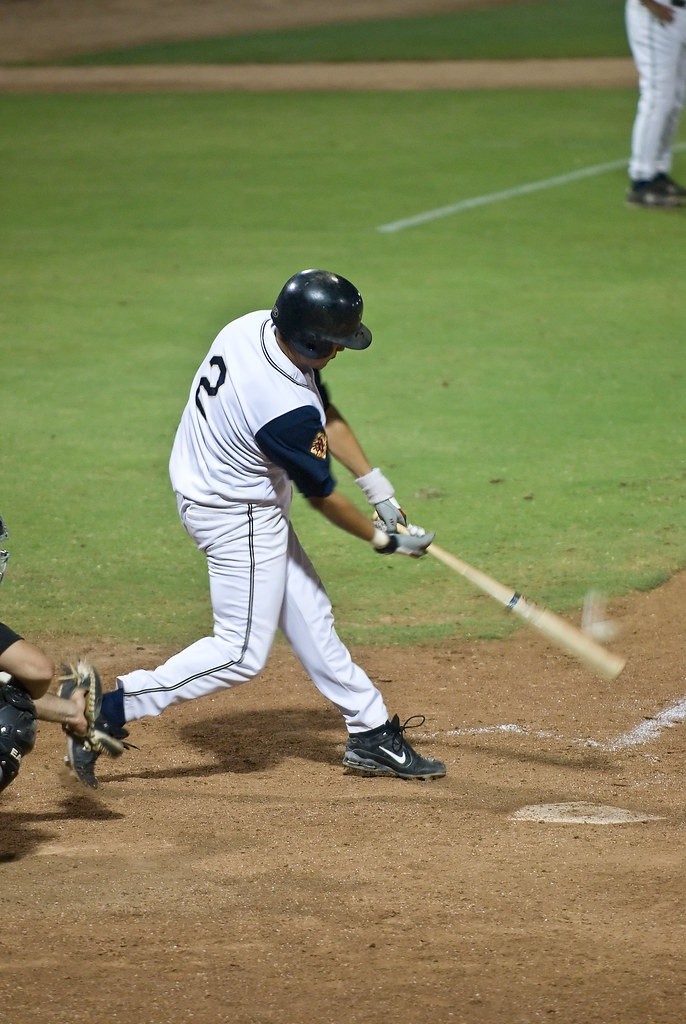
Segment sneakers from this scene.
[59,679,101,793]
[341,715,448,784]
[625,170,686,207]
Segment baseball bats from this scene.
[374,507,627,682]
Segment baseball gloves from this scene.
[52,657,130,760]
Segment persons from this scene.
[0,515,89,793]
[57,270,446,780]
[619,0,686,210]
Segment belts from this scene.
[672,0,686,8]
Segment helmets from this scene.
[269,268,377,358]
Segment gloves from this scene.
[354,466,409,538]
[368,526,438,559]
